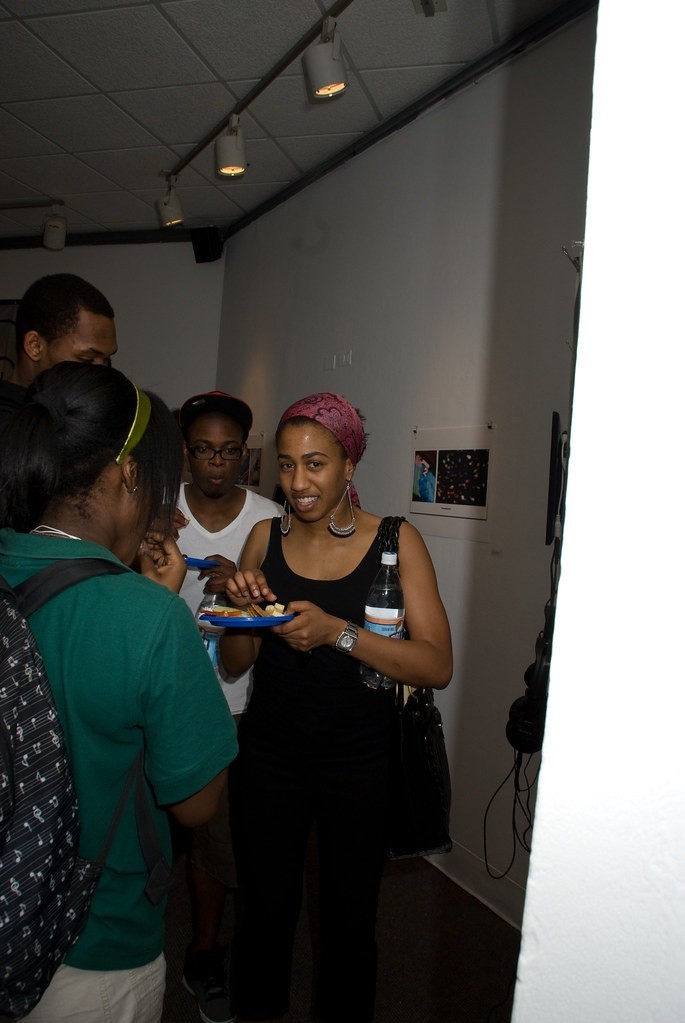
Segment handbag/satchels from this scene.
[399,686,452,852]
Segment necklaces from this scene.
[28,524,83,541]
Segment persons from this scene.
[0,357,240,1023]
[131,390,288,1023]
[219,391,456,1023]
[0,274,119,387]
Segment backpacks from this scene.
[0,557,170,1023]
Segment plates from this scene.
[184,556,221,570]
[198,614,294,627]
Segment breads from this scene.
[199,606,249,617]
[265,603,286,617]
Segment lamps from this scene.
[214,113,248,177]
[158,174,185,228]
[44,203,67,250]
[303,16,348,101]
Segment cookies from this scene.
[247,603,270,616]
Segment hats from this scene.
[179,391,253,444]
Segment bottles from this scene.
[359,550,406,691]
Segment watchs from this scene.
[334,621,358,653]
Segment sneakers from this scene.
[182,939,238,1023]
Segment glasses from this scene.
[186,444,244,461]
